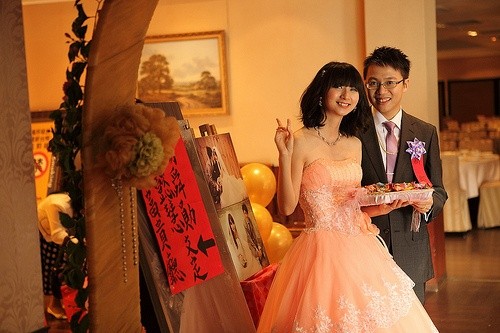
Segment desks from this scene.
[440,114,500,200]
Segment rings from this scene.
[420,204,427,209]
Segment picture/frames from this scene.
[136,29,230,117]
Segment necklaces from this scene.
[377,129,399,154]
[317,122,342,147]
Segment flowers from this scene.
[405,137,433,190]
[91,102,175,191]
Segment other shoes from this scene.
[46,306,68,319]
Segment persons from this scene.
[352,46,448,306]
[206,146,247,208]
[38,194,86,319]
[226,203,270,282]
[271,61,415,333]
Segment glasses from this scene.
[365,77,407,89]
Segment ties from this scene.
[382,121,399,184]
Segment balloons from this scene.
[264,222,293,264]
[249,203,273,244]
[240,163,276,208]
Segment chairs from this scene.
[440,152,472,238]
[478,183,500,228]
